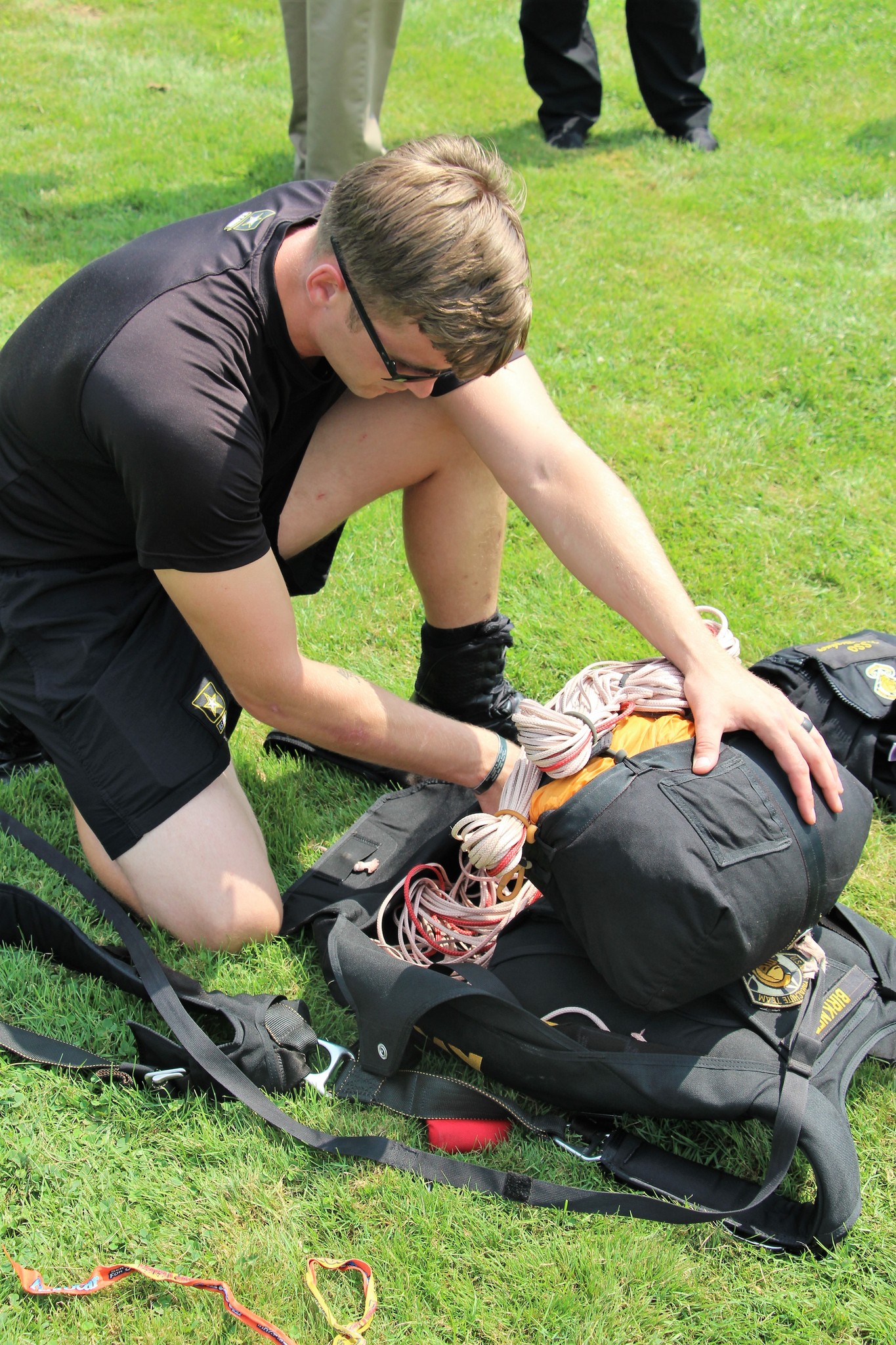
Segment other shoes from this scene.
[684,126,720,150]
[547,128,586,149]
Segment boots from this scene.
[408,608,522,747]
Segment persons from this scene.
[518,0,722,154]
[277,0,407,182]
[0,134,846,956]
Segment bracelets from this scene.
[470,727,507,796]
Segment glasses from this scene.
[329,235,458,382]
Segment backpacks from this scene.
[749,629,896,809]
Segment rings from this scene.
[799,716,813,734]
